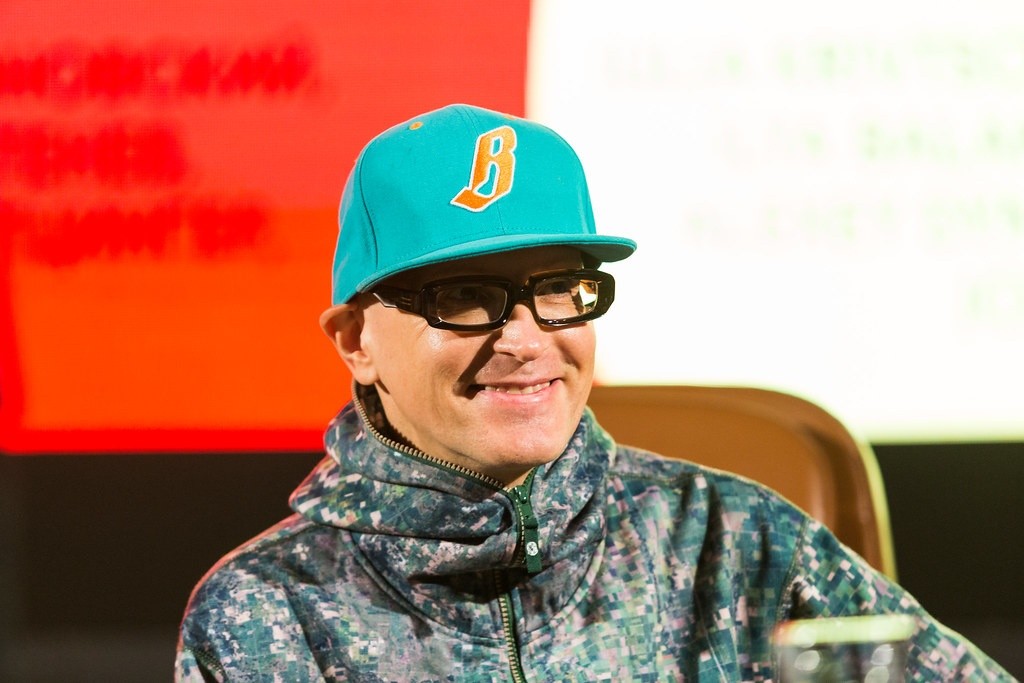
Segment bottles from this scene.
[772,614,916,682]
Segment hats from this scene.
[331,104,637,306]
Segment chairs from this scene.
[588,385,898,582]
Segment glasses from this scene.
[370,269,616,332]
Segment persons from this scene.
[173,104,1020,683]
